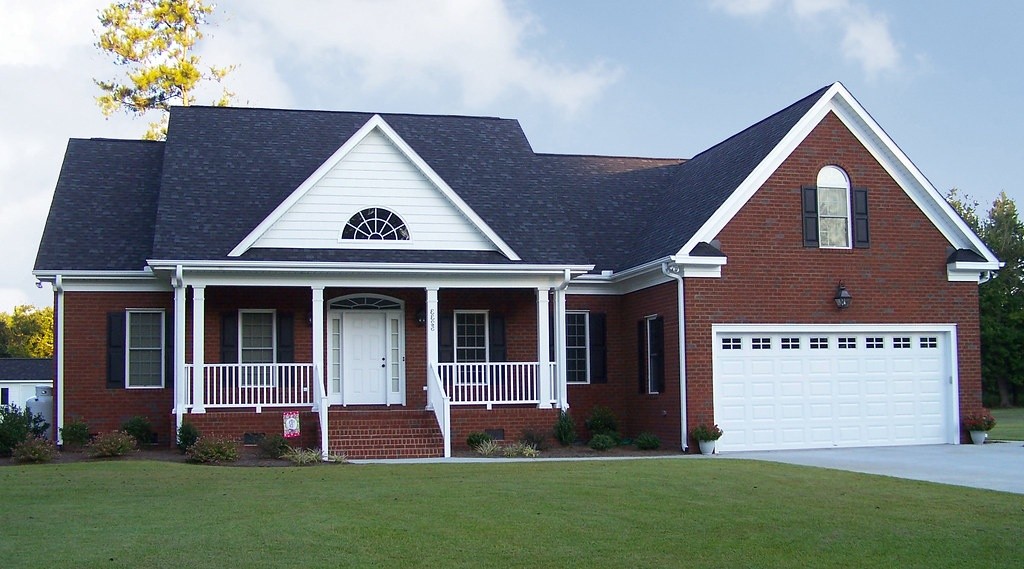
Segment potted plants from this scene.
[964,408,996,444]
[690,422,724,455]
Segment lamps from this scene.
[416,309,426,326]
[833,278,852,308]
